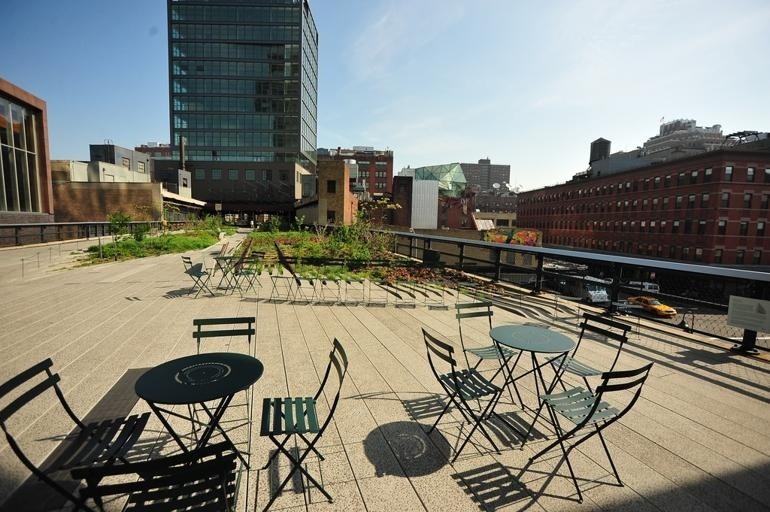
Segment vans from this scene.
[625,282,660,293]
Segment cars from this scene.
[628,295,677,317]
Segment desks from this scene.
[212,256,242,295]
[489,325,577,451]
[135,352,264,470]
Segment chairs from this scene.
[0,357,152,512]
[422,328,505,463]
[192,317,255,470]
[268,256,484,312]
[455,301,519,411]
[545,313,631,401]
[260,337,348,512]
[181,256,215,299]
[529,361,655,504]
[126,444,238,512]
[236,251,265,295]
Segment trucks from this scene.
[560,278,608,302]
